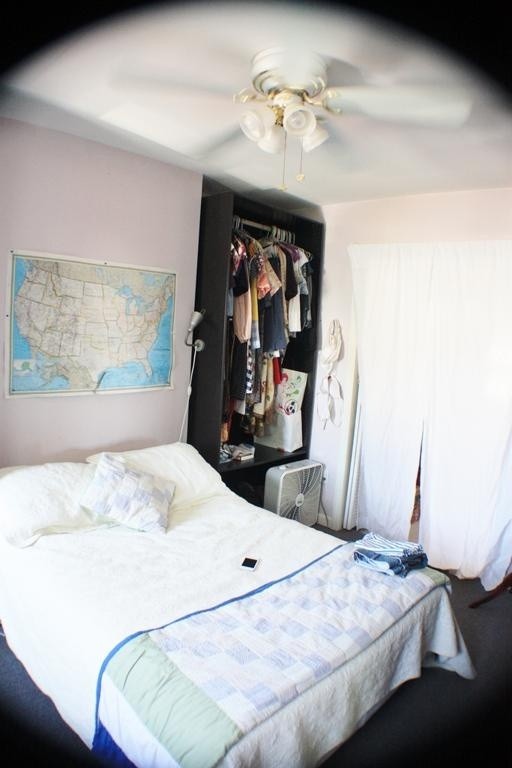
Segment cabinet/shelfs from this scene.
[188,190,326,472]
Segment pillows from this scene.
[92,442,226,513]
[1,462,116,547]
[85,459,177,535]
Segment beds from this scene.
[1,442,478,768]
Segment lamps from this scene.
[185,311,205,352]
[238,92,332,165]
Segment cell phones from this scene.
[240,556,258,571]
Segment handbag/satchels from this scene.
[253,410,308,453]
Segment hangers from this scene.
[232,216,304,260]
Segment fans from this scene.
[264,459,322,525]
[131,20,471,163]
